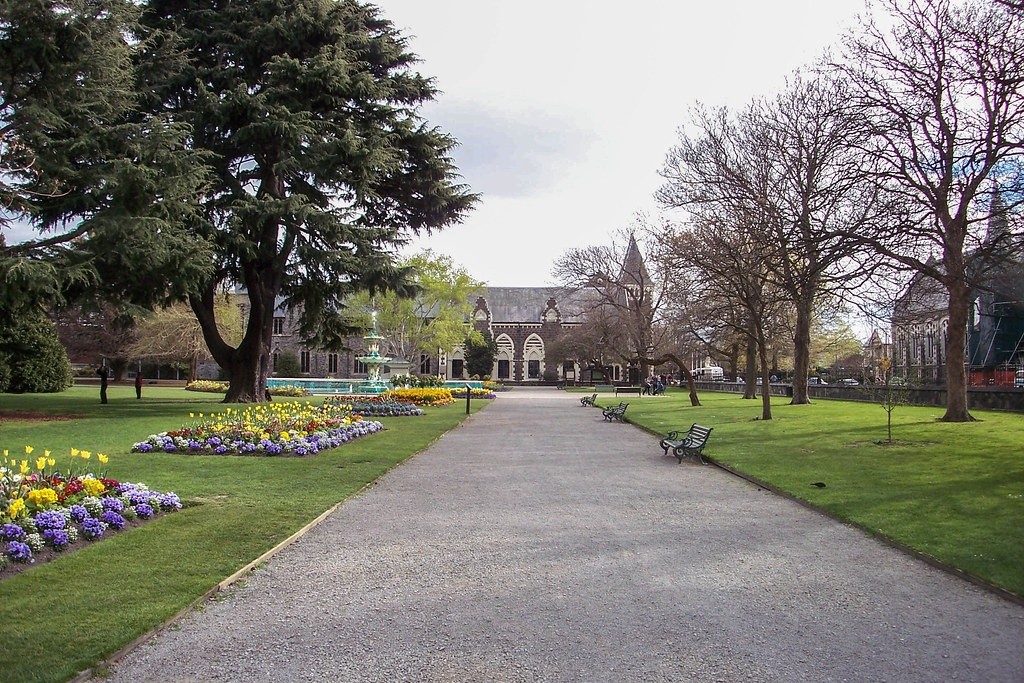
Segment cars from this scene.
[808,377,829,385]
[838,379,860,386]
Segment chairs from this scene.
[658,387,666,396]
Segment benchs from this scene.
[660,422,714,465]
[580,394,598,407]
[603,401,630,422]
[614,386,642,398]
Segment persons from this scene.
[134,372,142,399]
[97,365,108,404]
[642,376,651,395]
[653,381,664,395]
[651,374,661,390]
[770,373,777,383]
[817,377,821,384]
[668,374,672,385]
